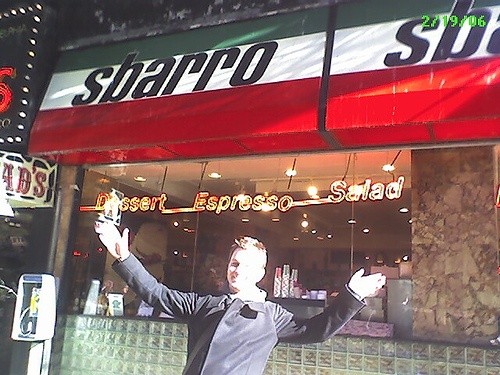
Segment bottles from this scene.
[273,264,298,299]
[83,280,100,315]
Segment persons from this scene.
[95,220,387,375]
